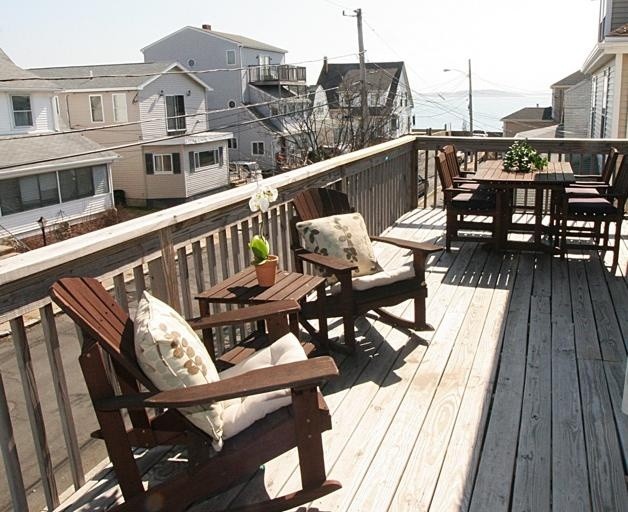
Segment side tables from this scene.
[195,263,327,391]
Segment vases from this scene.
[253,255,279,287]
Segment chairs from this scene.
[50,278,342,512]
[435,144,628,265]
[293,189,443,367]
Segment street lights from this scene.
[444,59,473,133]
[37,216,47,245]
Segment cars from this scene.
[415,175,429,199]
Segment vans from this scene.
[230,160,263,186]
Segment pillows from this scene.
[296,211,383,290]
[133,290,225,452]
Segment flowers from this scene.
[248,186,279,266]
